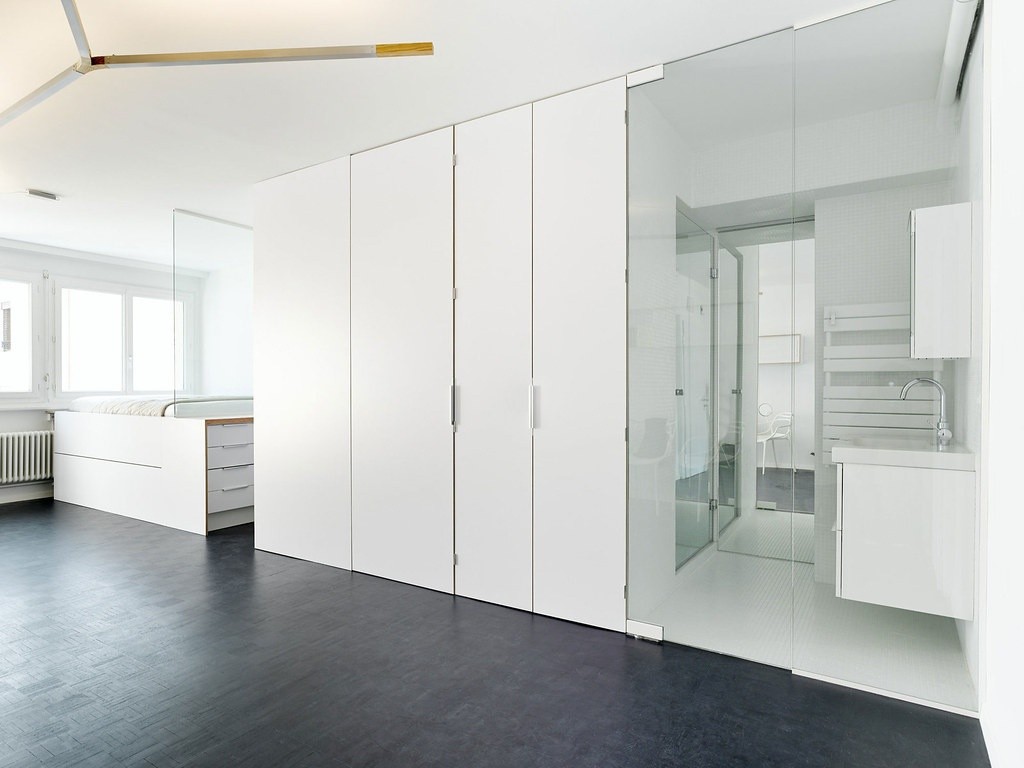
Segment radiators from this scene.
[0,430,54,484]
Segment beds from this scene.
[53,392,254,537]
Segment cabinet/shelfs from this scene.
[906,202,972,360]
[242,126,453,593]
[831,462,977,621]
[453,74,625,634]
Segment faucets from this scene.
[899,377,953,446]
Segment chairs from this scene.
[757,412,797,476]
[719,421,747,472]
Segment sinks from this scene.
[832,434,975,472]
